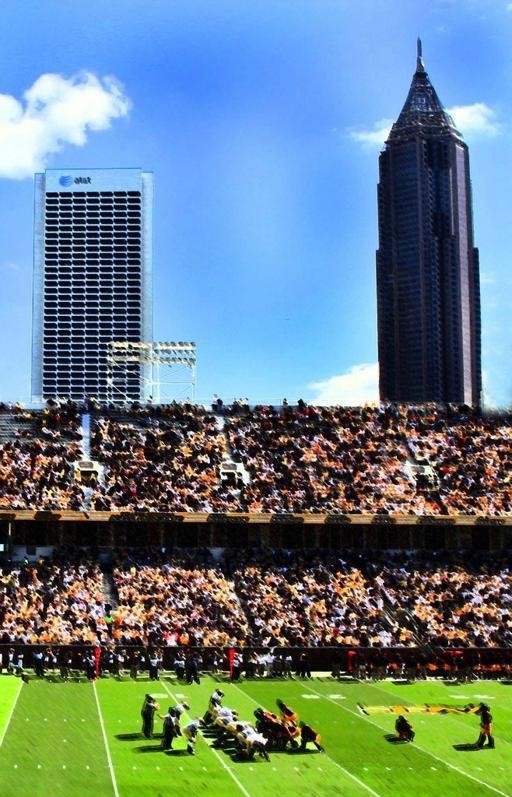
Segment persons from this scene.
[162,708,177,750]
[157,701,189,718]
[183,717,207,754]
[200,688,323,761]
[141,694,158,739]
[472,700,495,748]
[173,709,183,736]
[394,715,415,741]
